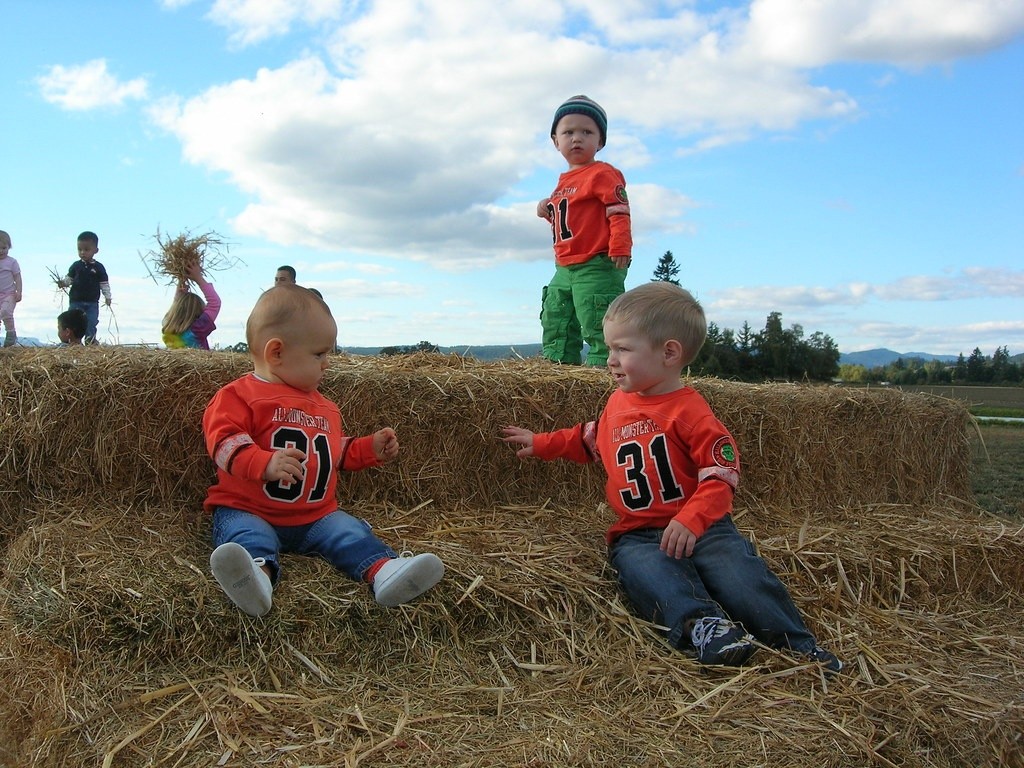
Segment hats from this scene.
[551,95,607,150]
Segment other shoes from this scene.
[372,552,444,607]
[209,542,272,618]
[4,331,16,346]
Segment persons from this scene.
[57,308,88,344]
[502,281,844,681]
[275,266,296,286]
[58,232,112,345]
[538,96,632,365]
[202,284,444,616]
[160,259,220,350]
[0,231,22,347]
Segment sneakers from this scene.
[806,645,843,678]
[686,605,757,669]
